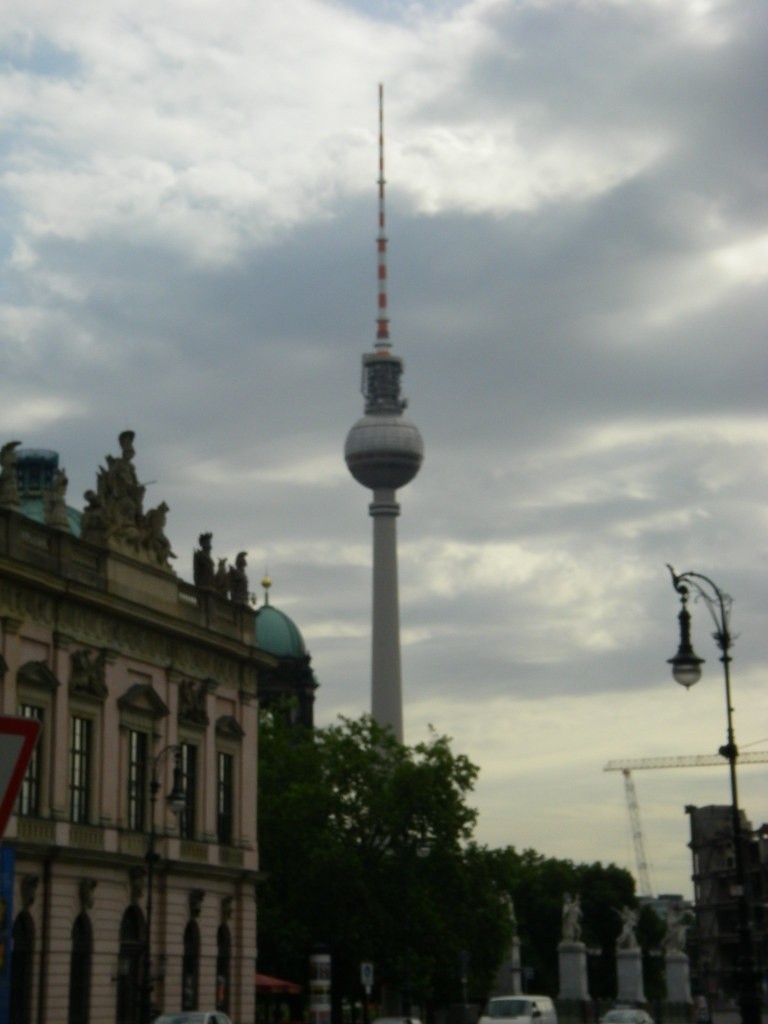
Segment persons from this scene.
[79,431,250,603]
[0,442,24,505]
[561,894,583,942]
[664,896,697,952]
[617,907,641,949]
[43,470,69,525]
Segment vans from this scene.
[477,995,557,1024]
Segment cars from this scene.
[152,1010,234,1024]
[369,1016,424,1024]
[598,1009,655,1024]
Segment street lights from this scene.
[667,570,765,1024]
